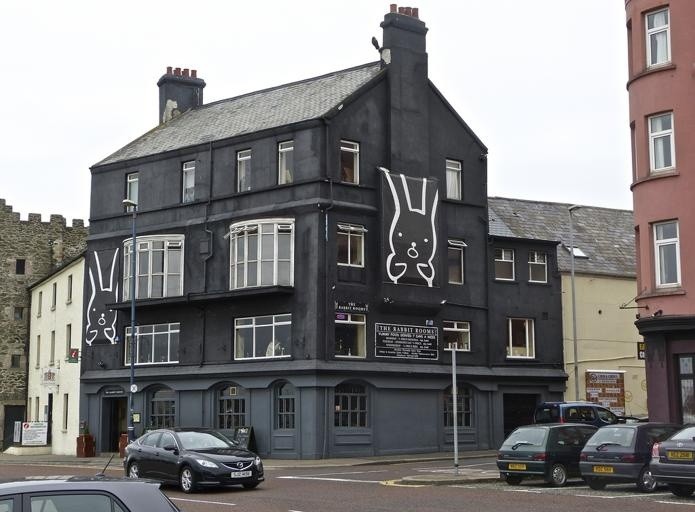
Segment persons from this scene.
[265,337,282,357]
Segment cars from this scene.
[123,428,264,494]
[0,455,179,512]
[496,402,695,499]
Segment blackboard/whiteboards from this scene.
[233,426,252,449]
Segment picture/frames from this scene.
[133,413,141,423]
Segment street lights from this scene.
[568,204,582,401]
[120,200,137,446]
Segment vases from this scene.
[80,426,88,433]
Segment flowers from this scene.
[80,421,87,429]
